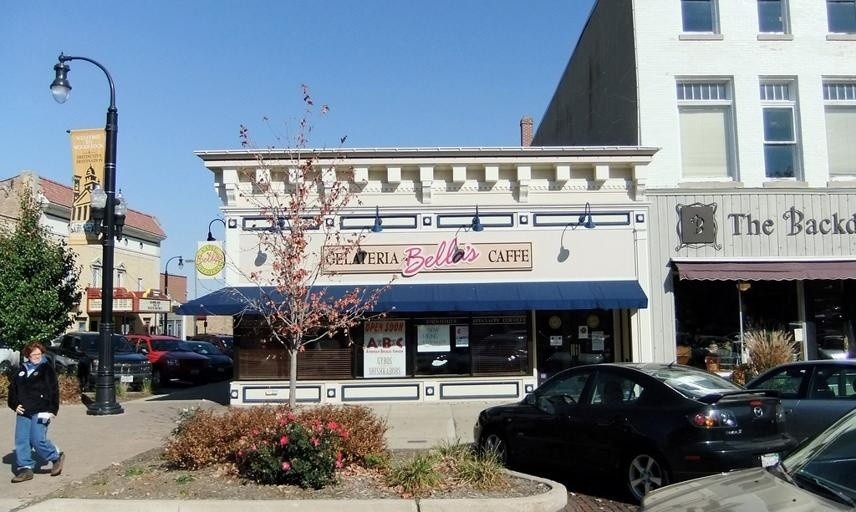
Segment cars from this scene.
[819,334,848,360]
[431,333,526,373]
[695,336,743,370]
[640,403,854,511]
[475,361,801,505]
[739,362,856,440]
[0,332,232,390]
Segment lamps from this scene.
[273,203,594,231]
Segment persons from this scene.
[5,340,66,484]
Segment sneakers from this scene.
[11,469,33,483]
[50,451,65,476]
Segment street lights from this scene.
[163,256,184,335]
[51,53,126,415]
